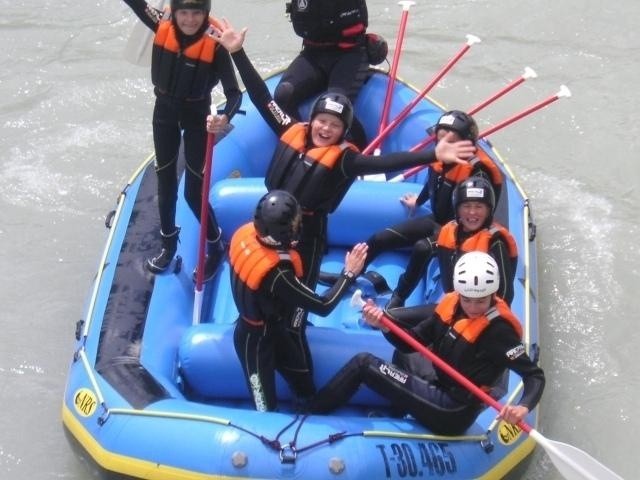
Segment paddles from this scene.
[192,106,218,327]
[349,290,626,480]
[364,0,417,182]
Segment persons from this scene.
[295,252,545,437]
[207,17,476,327]
[318,110,502,331]
[123,0,242,284]
[230,190,369,413]
[274,0,368,153]
[382,177,518,330]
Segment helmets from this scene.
[172,0,210,13]
[256,191,302,248]
[453,251,499,297]
[310,92,355,132]
[453,178,495,213]
[437,111,479,147]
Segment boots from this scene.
[318,270,346,287]
[150,230,178,273]
[194,228,226,281]
[384,274,415,311]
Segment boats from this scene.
[63,62,540,480]
